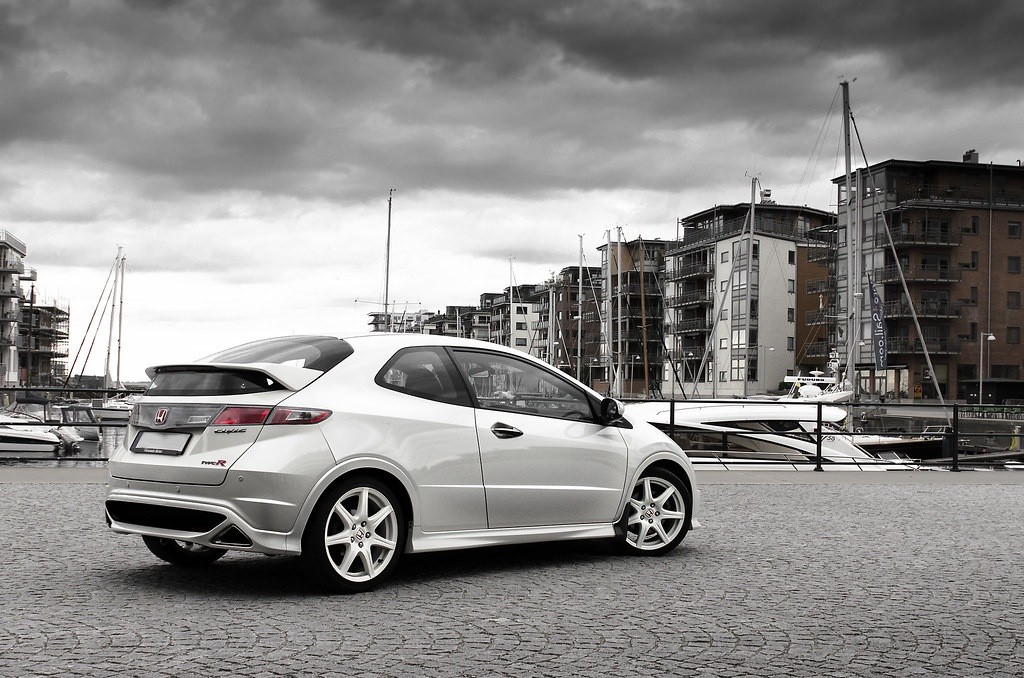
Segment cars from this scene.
[103,331,701,593]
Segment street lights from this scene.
[980,332,996,404]
[682,350,693,390]
[764,344,775,392]
[846,338,865,371]
[589,357,598,389]
[631,354,641,397]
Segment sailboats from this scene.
[491,81,965,469]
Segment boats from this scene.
[0,397,137,452]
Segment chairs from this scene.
[405,367,445,397]
